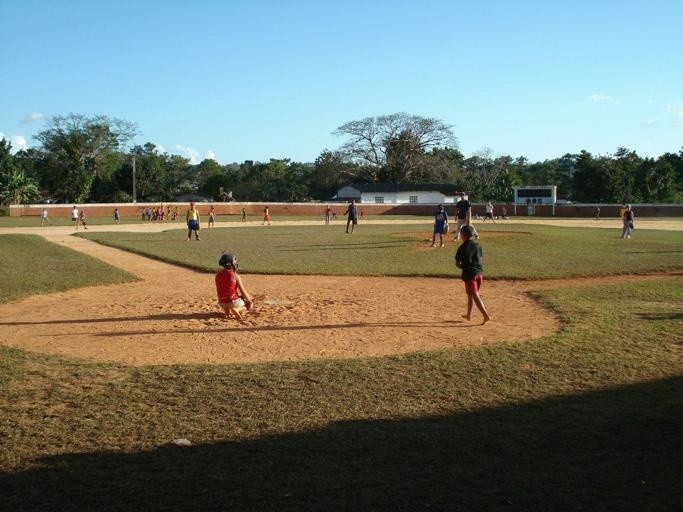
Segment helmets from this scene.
[219,254,240,271]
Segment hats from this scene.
[460,191,467,196]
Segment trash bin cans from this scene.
[527,206,535,216]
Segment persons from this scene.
[344,200,357,234]
[141,205,177,222]
[431,204,450,248]
[71,206,79,225]
[112,208,120,224]
[241,208,247,222]
[261,206,271,225]
[208,205,215,227]
[455,224,491,325]
[596,207,600,218]
[619,203,626,224]
[325,205,331,224]
[215,253,253,320]
[452,191,471,241]
[621,204,634,239]
[186,201,201,241]
[483,200,506,224]
[76,210,88,229]
[40,208,48,225]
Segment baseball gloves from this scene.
[444,226,449,235]
